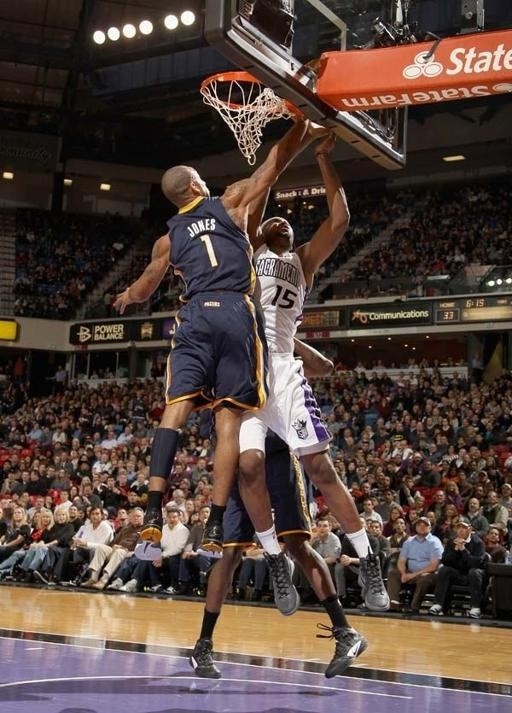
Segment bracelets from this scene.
[314,149,329,158]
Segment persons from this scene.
[113,117,309,552]
[136,254,152,278]
[137,265,185,314]
[12,209,136,319]
[261,196,331,249]
[238,131,390,615]
[2,355,510,616]
[346,181,510,236]
[312,231,510,299]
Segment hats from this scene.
[412,517,430,526]
[455,517,471,527]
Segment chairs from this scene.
[64,375,166,393]
[326,361,469,383]
[261,184,508,303]
[203,526,510,620]
[2,205,183,317]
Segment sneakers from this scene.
[140,508,161,543]
[428,604,444,616]
[316,622,368,678]
[7,570,138,592]
[189,638,221,678]
[200,523,223,553]
[357,546,390,611]
[262,551,300,615]
[144,584,205,597]
[469,608,482,619]
